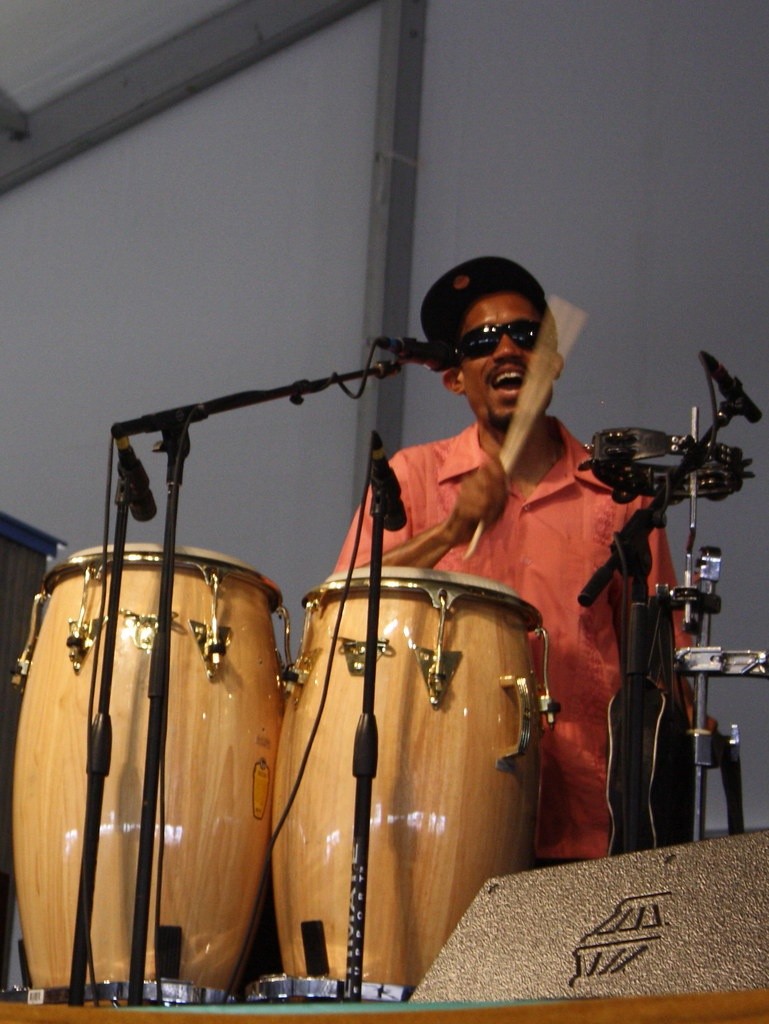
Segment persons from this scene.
[329,256,717,874]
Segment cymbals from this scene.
[576,428,756,505]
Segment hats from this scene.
[421,256,558,352]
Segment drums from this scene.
[271,566,544,999]
[13,543,288,1006]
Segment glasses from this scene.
[454,319,542,358]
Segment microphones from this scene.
[376,337,460,372]
[113,428,156,521]
[369,432,408,532]
[699,351,763,424]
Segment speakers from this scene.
[407,829,769,1005]
